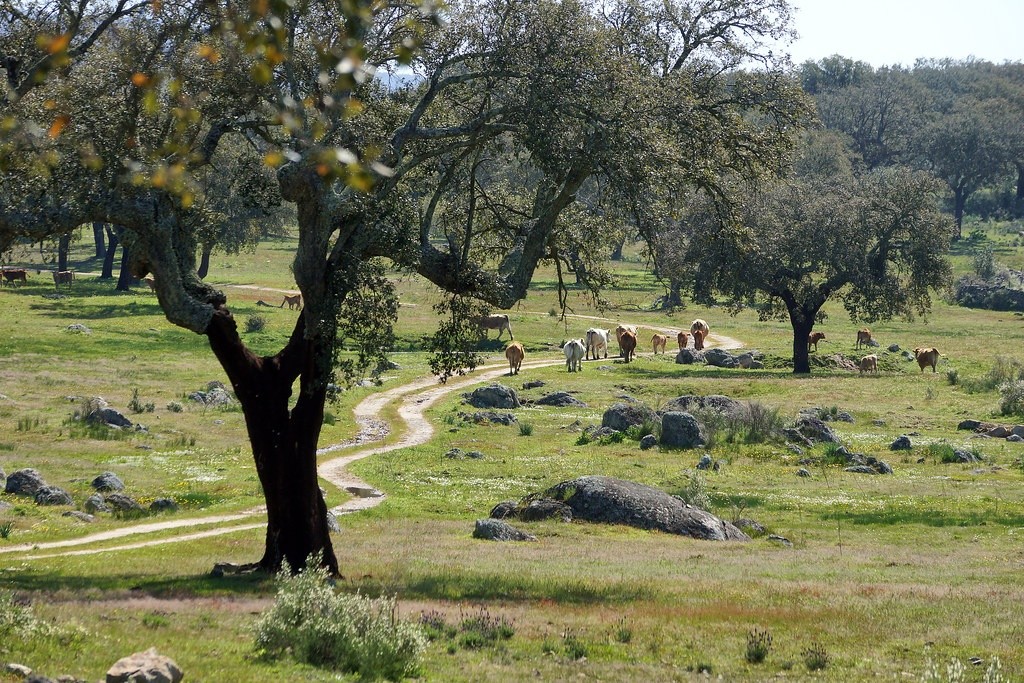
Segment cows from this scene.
[0,270,75,291]
[467,314,709,376]
[807,328,942,373]
[284,295,301,310]
